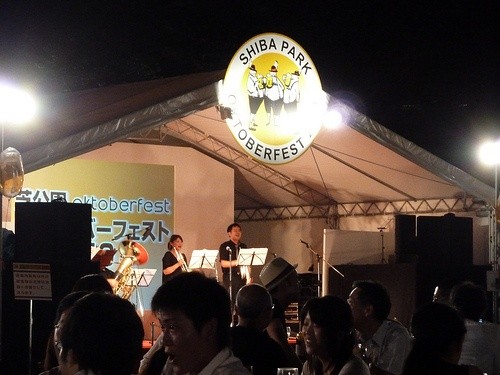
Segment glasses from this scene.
[347,299,361,305]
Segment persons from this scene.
[0,224,499,375]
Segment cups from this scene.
[277,368,298,375]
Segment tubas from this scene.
[109,239,149,300]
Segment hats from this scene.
[258,256,298,290]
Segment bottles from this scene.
[295,331,307,362]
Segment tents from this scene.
[0,66,499,325]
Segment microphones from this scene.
[225,245,233,254]
